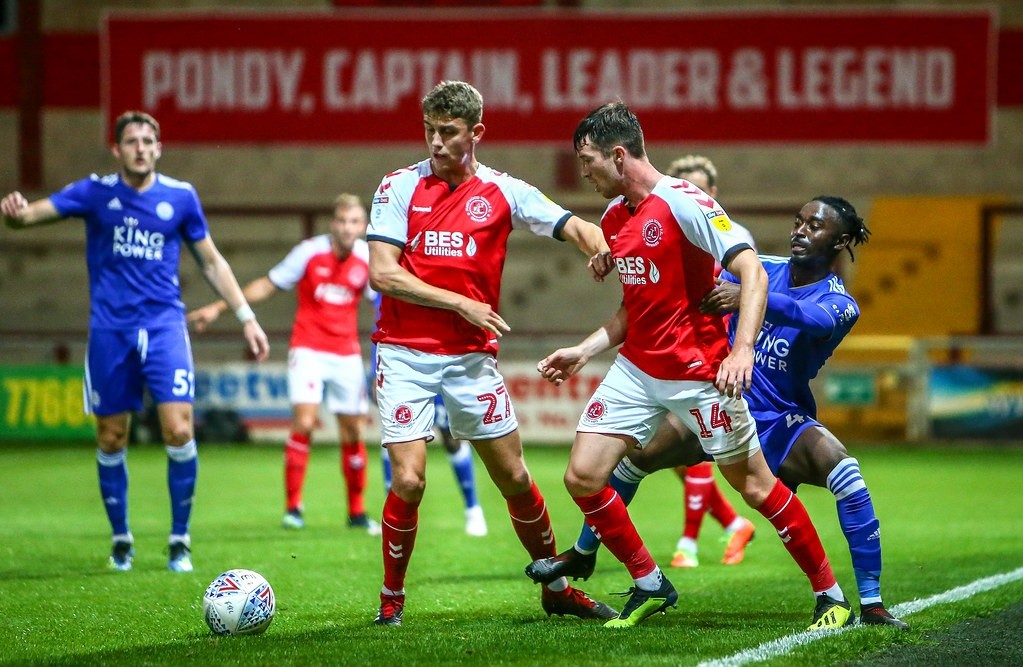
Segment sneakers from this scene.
[283,507,303,530]
[807,592,855,634]
[603,572,678,629]
[107,538,134,571]
[721,517,755,565]
[347,518,382,537]
[540,583,620,620]
[163,542,193,574]
[373,591,405,629]
[859,602,908,631]
[670,537,699,569]
[525,546,597,586]
[465,506,487,537]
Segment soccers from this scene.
[203,569,276,635]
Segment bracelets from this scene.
[234,305,256,324]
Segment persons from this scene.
[185,194,370,529]
[526,156,910,625]
[363,79,620,627]
[0,110,270,571]
[564,99,855,633]
[367,394,484,537]
